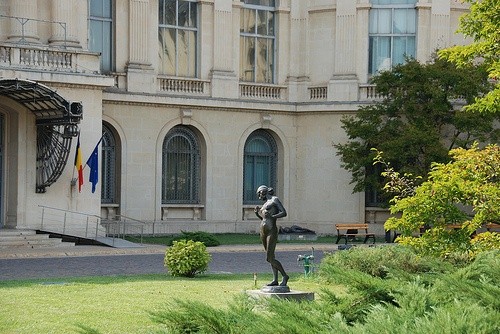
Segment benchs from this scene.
[335,224,376,245]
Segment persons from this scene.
[254,184,290,286]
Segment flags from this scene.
[86,146,98,193]
[74,137,83,192]
